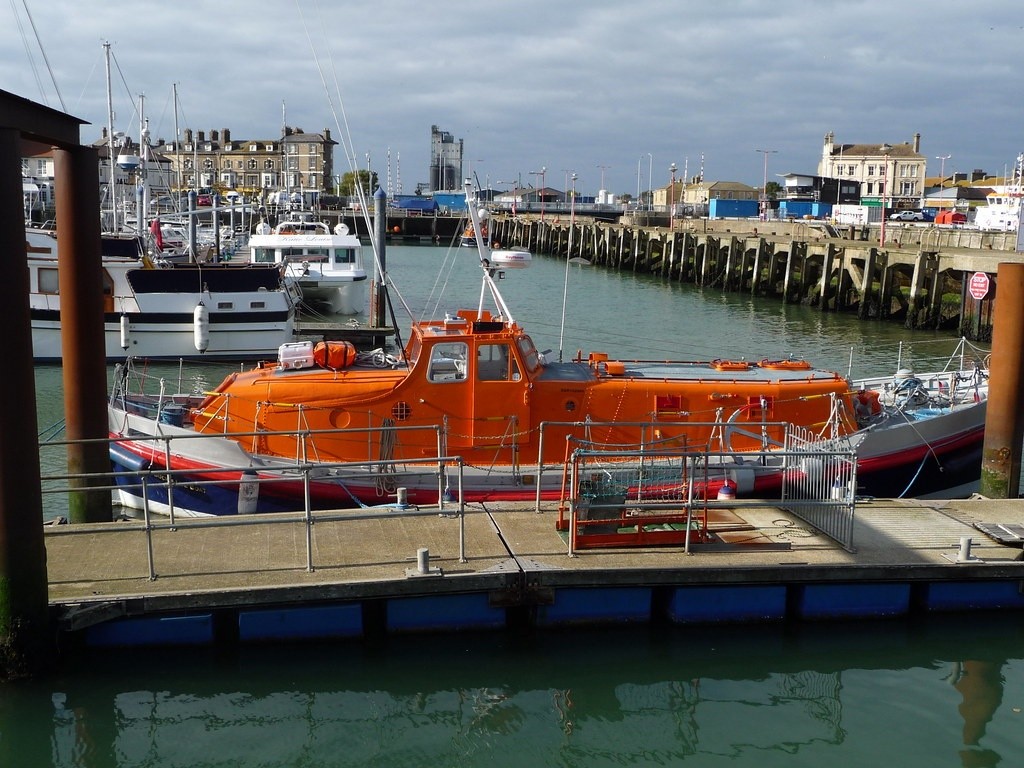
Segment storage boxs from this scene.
[710,199,833,218]
[394,194,436,211]
[278,341,315,372]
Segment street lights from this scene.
[755,149,777,221]
[647,152,653,212]
[596,165,611,191]
[442,156,484,191]
[667,163,678,231]
[497,180,519,217]
[570,173,579,225]
[560,168,576,206]
[936,152,952,214]
[527,166,546,222]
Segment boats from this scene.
[247,215,367,318]
[105,168,988,523]
[26,232,329,366]
[459,217,489,250]
[491,250,532,270]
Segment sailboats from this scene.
[92,43,245,262]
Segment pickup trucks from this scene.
[890,210,924,222]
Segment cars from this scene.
[227,191,240,202]
[197,194,213,206]
[150,195,171,205]
